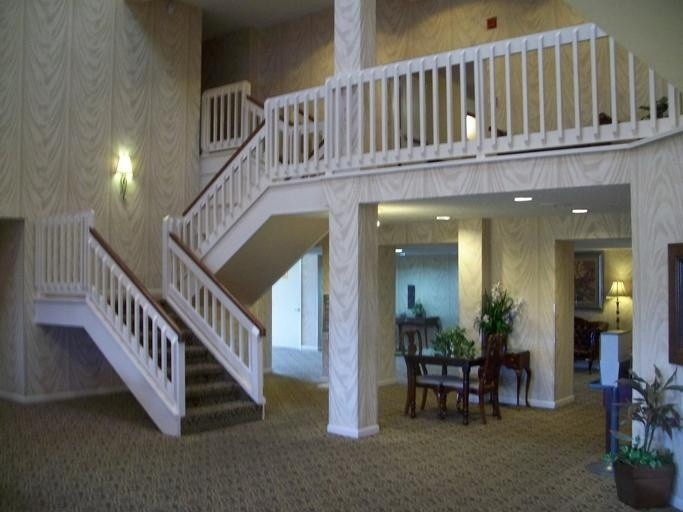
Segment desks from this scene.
[588,373,631,476]
[496,349,533,409]
[394,316,440,349]
[405,348,493,425]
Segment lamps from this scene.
[112,148,133,201]
[604,280,631,332]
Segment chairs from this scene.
[437,332,503,422]
[399,329,448,420]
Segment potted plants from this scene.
[431,326,474,359]
[609,365,682,508]
[411,303,425,319]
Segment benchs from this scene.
[574,315,608,375]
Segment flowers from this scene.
[474,280,522,331]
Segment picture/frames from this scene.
[573,251,606,311]
